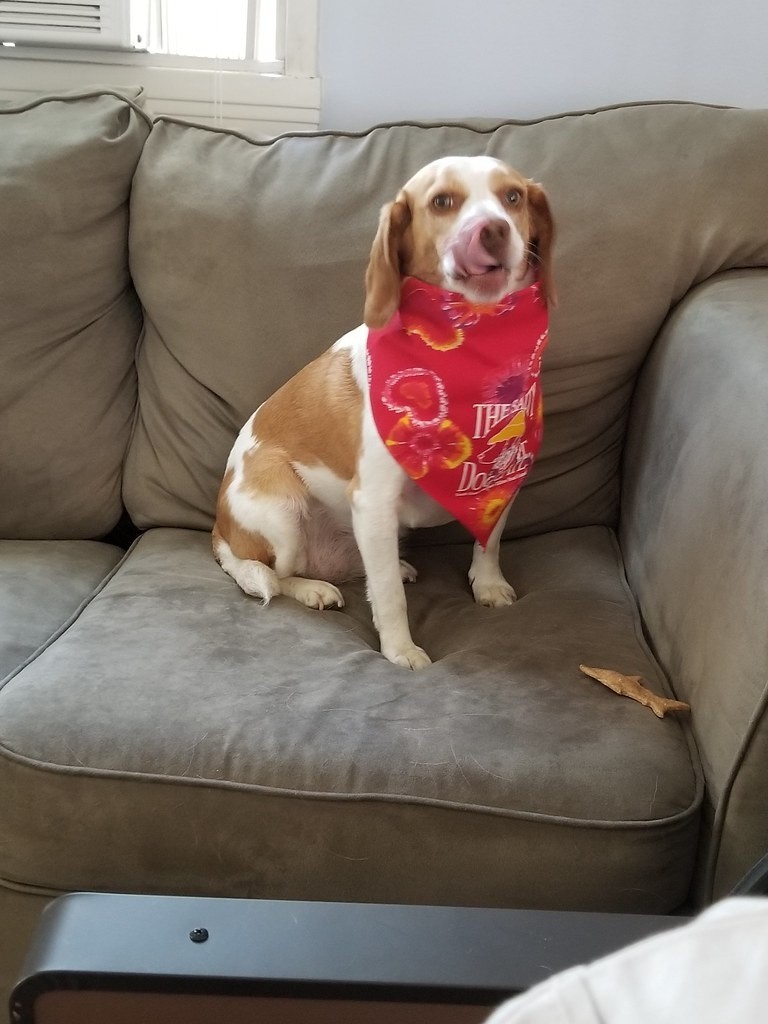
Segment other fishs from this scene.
[580,665,690,718]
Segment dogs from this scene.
[214,157,553,667]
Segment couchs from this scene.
[0,86,768,1024]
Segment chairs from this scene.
[9,850,768,1023]
